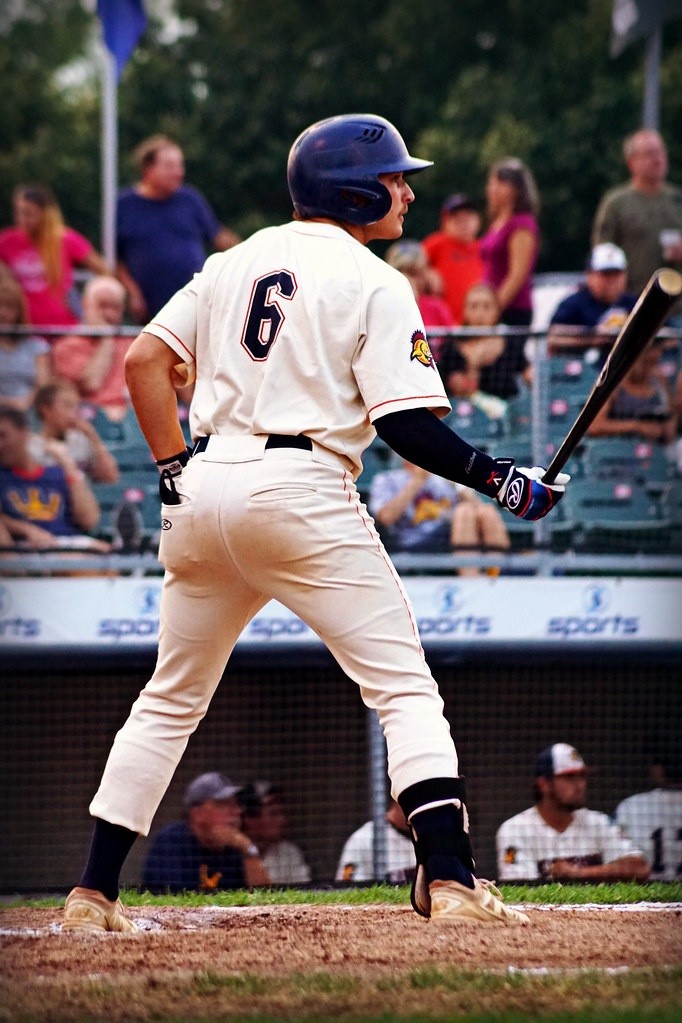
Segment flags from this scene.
[607,0,674,77]
[94,0,146,84]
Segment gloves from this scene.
[153,445,194,491]
[474,456,571,521]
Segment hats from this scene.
[185,772,242,803]
[538,742,597,779]
[237,782,282,805]
[590,243,627,271]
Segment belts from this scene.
[194,432,312,452]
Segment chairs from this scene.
[84,350,681,554]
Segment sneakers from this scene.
[428,874,531,926]
[63,887,140,934]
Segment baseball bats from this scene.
[540,268,682,486]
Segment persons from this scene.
[334,802,419,883]
[371,128,682,576]
[496,739,682,883]
[139,771,311,893]
[61,114,570,929]
[0,136,242,583]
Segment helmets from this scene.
[287,114,434,225]
